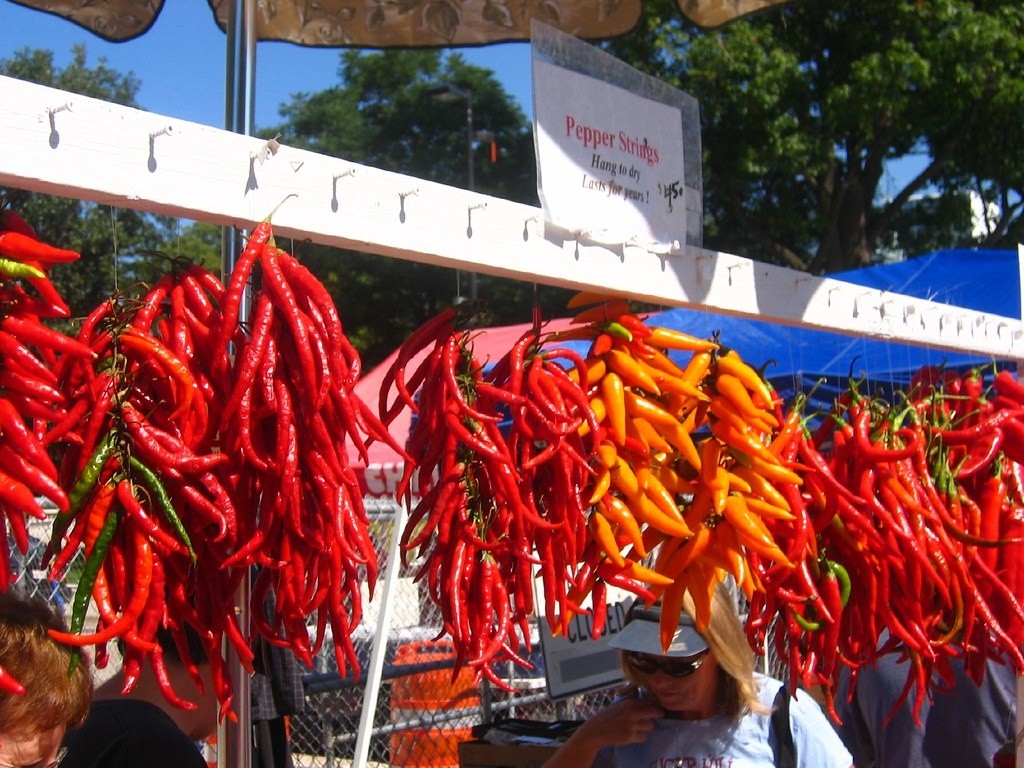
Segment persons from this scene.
[541,583,856,768]
[58,600,218,768]
[0,587,95,768]
[826,625,1016,768]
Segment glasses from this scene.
[629,651,709,677]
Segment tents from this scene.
[346,308,663,768]
[407,249,1021,716]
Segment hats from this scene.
[607,601,710,658]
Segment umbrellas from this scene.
[14,0,785,768]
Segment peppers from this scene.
[0,192,1024,728]
[993,745,1016,768]
